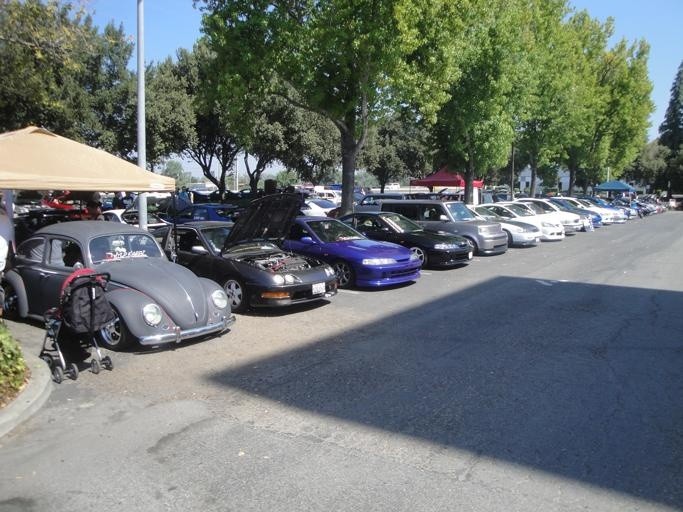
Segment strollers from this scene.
[40,267,115,382]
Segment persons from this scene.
[111,191,124,209]
[173,186,191,203]
[81,201,104,222]
[123,191,135,210]
